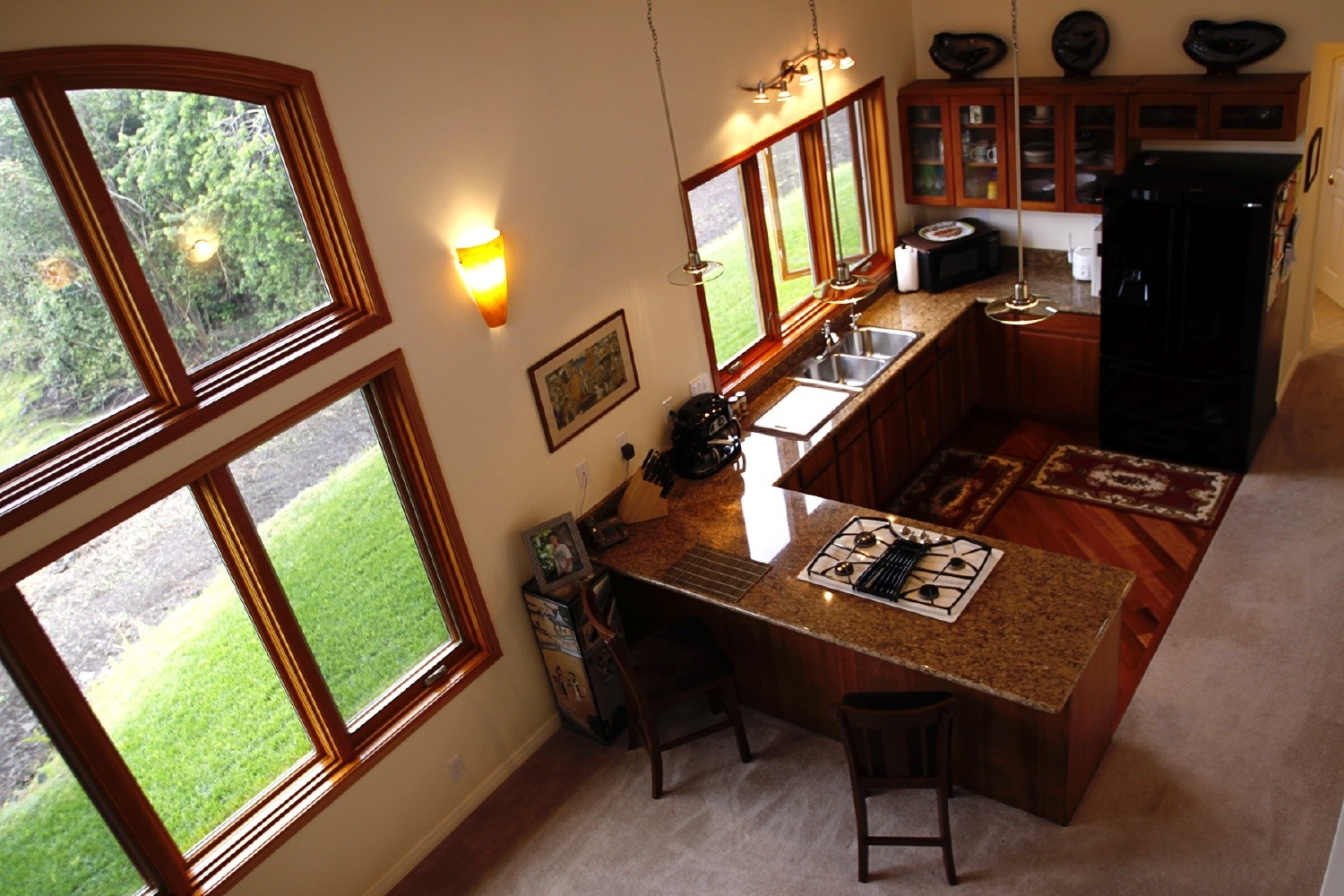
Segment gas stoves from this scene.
[795,514,1004,625]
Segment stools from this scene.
[830,690,957,886]
[579,583,745,798]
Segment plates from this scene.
[919,221,975,240]
[1052,9,1111,70]
[1076,173,1097,188]
[1029,116,1054,123]
[1022,179,1050,191]
[1075,138,1113,163]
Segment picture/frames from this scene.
[519,511,594,594]
[591,515,630,551]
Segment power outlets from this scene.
[689,372,710,396]
[614,430,631,459]
[526,308,640,453]
[911,214,920,232]
[663,396,676,423]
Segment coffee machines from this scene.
[669,393,743,479]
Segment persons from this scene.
[547,532,573,577]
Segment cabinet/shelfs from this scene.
[1005,75,1140,213]
[976,303,1101,426]
[781,307,987,508]
[897,78,1012,207]
[522,569,634,748]
[1131,72,1314,144]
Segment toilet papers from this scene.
[893,245,919,292]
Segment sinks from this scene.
[833,324,926,359]
[785,356,885,392]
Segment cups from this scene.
[1036,106,1050,116]
[939,131,996,163]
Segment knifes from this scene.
[641,448,675,498]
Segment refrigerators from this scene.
[1100,157,1305,476]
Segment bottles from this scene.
[736,391,747,414]
[988,172,997,200]
[728,396,742,421]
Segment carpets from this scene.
[1020,438,1244,530]
[887,443,1033,533]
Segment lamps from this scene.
[808,0,879,303]
[647,0,724,287]
[744,47,855,104]
[457,229,509,328]
[981,0,1058,327]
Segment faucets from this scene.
[814,318,850,360]
[849,312,863,331]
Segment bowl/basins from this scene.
[1021,141,1054,163]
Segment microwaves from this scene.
[899,218,1004,293]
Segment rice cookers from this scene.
[1072,245,1092,281]
